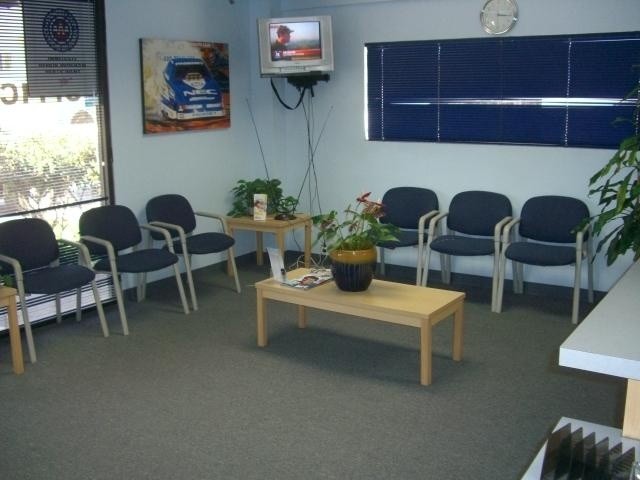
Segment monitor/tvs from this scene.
[256,15,334,80]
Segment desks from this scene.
[558,258,640,442]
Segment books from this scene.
[281,269,333,290]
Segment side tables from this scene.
[0,286,25,376]
[224,210,313,278]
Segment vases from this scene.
[329,242,376,292]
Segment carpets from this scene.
[521,416,640,480]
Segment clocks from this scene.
[480,0,519,36]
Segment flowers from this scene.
[310,191,401,252]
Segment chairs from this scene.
[76,204,190,335]
[366,187,446,286]
[495,195,594,325]
[1,219,109,364]
[422,191,517,313]
[142,194,242,310]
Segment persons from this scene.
[271,25,294,60]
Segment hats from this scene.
[276,25,294,33]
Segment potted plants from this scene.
[227,178,300,218]
